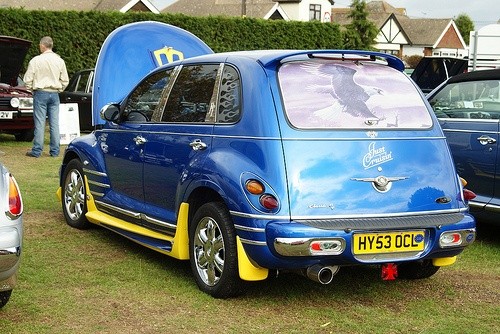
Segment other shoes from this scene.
[26,151,36,157]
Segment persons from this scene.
[23,36,69,157]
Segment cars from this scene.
[58,21,477,301]
[0,160,24,311]
[0,34,41,141]
[422,68,500,226]
